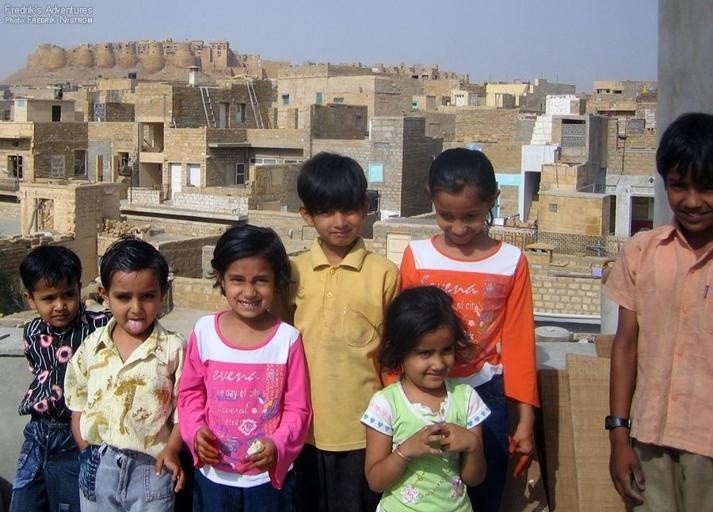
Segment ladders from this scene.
[247,79,265,129]
[200,87,216,128]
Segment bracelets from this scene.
[605,416,631,429]
[396,444,414,461]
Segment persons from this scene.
[602,112,713,512]
[359,285,492,512]
[177,224,313,512]
[9,245,112,512]
[268,151,401,511]
[380,148,541,512]
[62,236,188,512]
